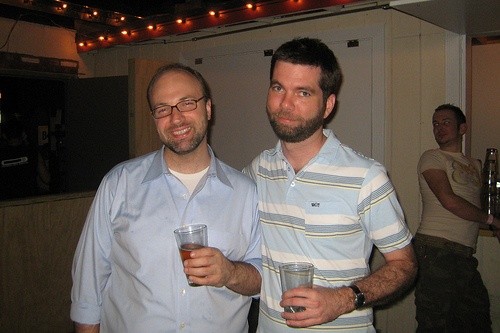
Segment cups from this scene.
[174,224,209,287]
[279,262,314,328]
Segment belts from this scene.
[413,233,474,258]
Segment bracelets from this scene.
[485,214,493,225]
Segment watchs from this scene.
[348,284,367,309]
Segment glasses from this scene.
[150,95,209,119]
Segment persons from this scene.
[69,63,264,333]
[234,37,419,332]
[414,103,500,333]
